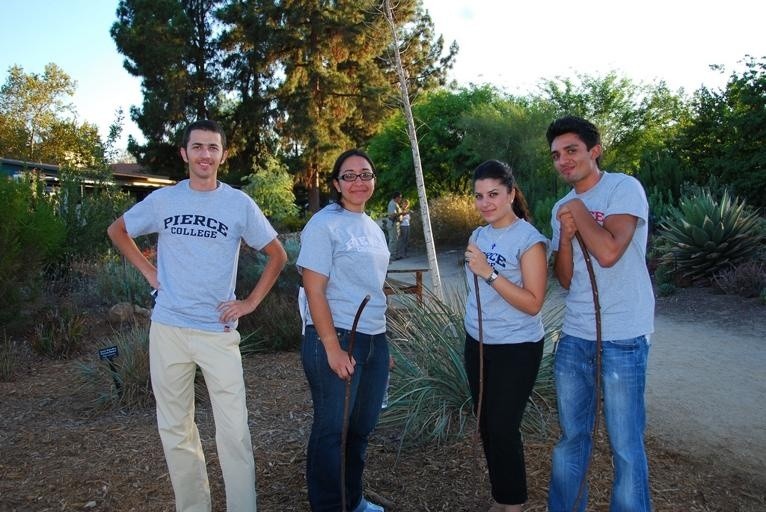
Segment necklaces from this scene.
[491,216,518,250]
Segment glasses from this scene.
[336,172,376,182]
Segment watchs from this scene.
[486,270,498,286]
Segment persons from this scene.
[296,149,396,511]
[463,160,551,511]
[387,191,408,261]
[108,119,289,511]
[545,116,656,511]
[400,200,411,257]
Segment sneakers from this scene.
[390,254,411,261]
[354,495,384,512]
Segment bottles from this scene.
[381,371,390,409]
